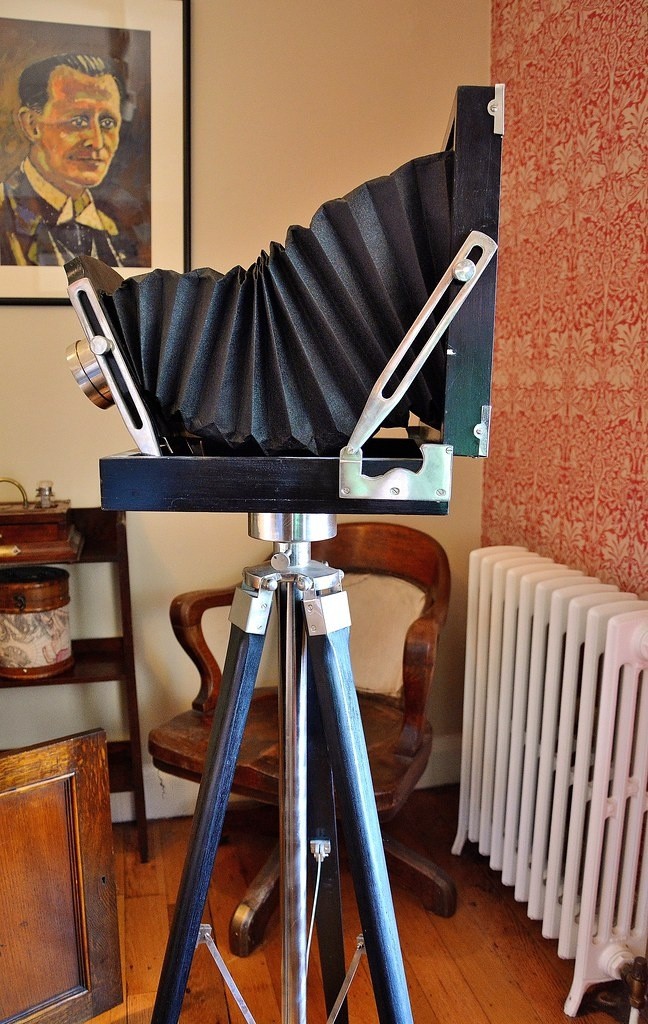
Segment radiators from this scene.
[448,544,648,1018]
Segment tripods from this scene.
[153,512,414,1024]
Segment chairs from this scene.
[136,522,458,958]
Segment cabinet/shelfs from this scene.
[0,501,148,869]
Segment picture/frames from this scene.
[0,0,191,307]
